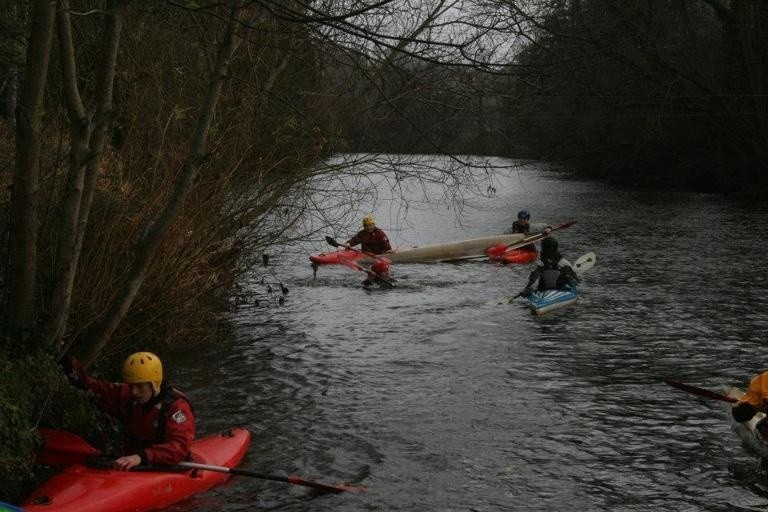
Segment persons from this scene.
[341,216,393,255]
[361,260,399,290]
[60,350,198,477]
[518,238,583,298]
[730,371,767,440]
[503,210,553,253]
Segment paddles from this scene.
[484,220,578,257]
[326,236,393,265]
[665,380,768,413]
[337,254,395,288]
[486,252,596,306]
[38,431,364,493]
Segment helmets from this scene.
[122,351,163,397]
[518,209,530,218]
[362,215,376,225]
[542,238,558,251]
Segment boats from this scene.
[21,427,250,512]
[724,386,768,445]
[309,233,578,316]
